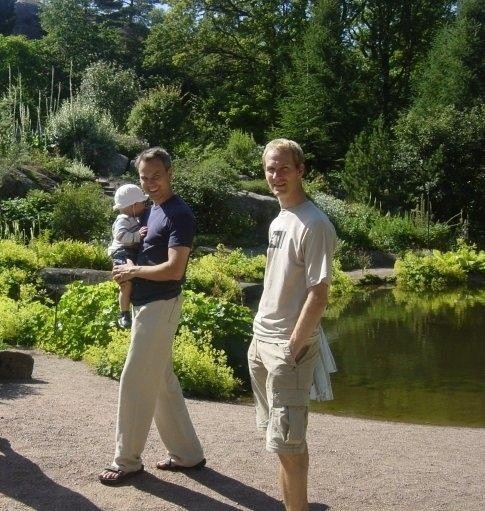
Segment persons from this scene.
[97,146,206,486]
[247,138,337,511]
[107,183,149,329]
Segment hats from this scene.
[112,183,150,212]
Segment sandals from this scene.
[98,464,144,485]
[156,456,206,470]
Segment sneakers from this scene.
[118,313,132,329]
